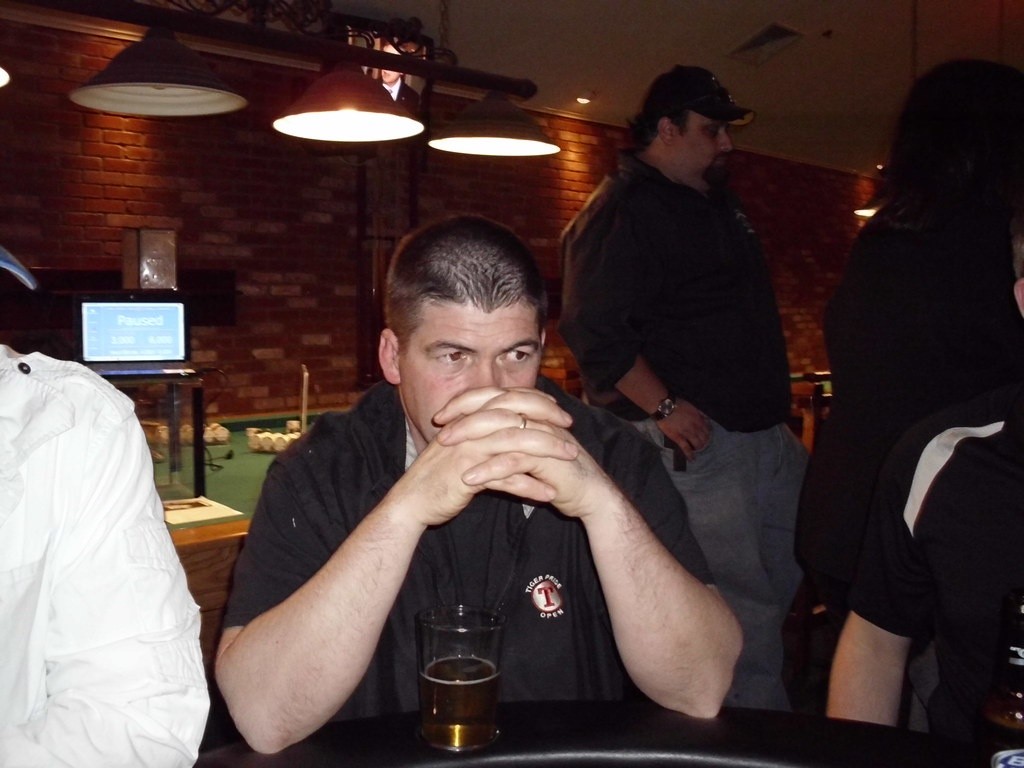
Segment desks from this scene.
[192,700,991,768]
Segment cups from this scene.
[415,604,508,752]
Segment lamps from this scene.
[270,62,424,142]
[68,27,250,118]
[426,90,561,157]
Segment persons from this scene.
[215,211,744,754]
[793,59,1024,738]
[0,344,211,768]
[556,66,808,710]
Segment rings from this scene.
[519,414,527,428]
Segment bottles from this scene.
[972,593,1024,768]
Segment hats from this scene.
[646,66,754,125]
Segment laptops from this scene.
[75,290,197,379]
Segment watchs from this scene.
[651,388,677,421]
